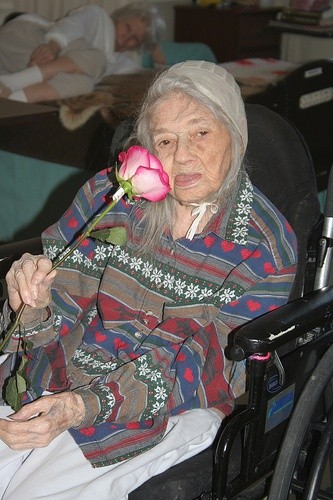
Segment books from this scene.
[285,5,333,18]
[277,10,333,28]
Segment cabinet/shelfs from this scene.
[172,5,284,60]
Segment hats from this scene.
[166,58,248,150]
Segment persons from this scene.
[0,0,168,108]
[0,58,301,500]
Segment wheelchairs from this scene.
[0,102,332,500]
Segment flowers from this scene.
[0,145,172,413]
